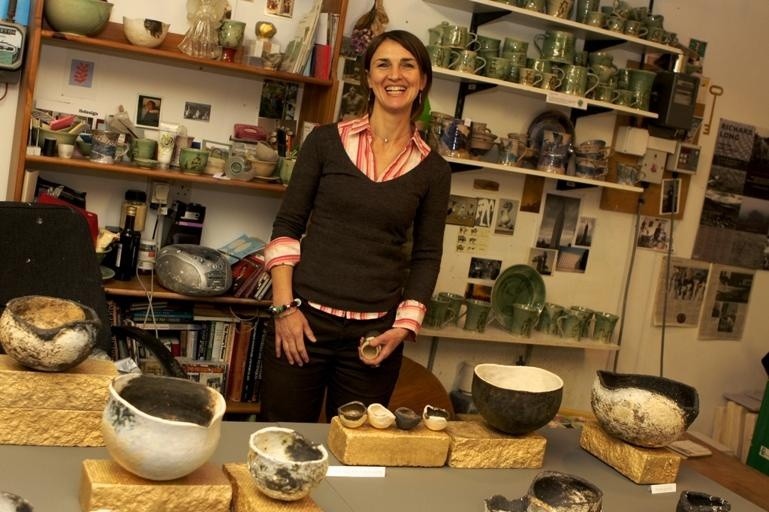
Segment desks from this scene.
[0,418,767,512]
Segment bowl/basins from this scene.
[469,358,568,439]
[248,426,329,503]
[0,296,104,374]
[122,14,174,51]
[40,0,115,44]
[583,365,695,452]
[98,369,229,484]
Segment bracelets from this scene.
[271,306,302,321]
[264,297,307,312]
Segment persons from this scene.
[139,98,162,125]
[256,29,453,424]
[339,85,371,120]
[718,303,739,332]
[473,198,493,227]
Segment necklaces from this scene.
[368,124,412,146]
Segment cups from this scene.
[415,109,648,185]
[485,466,603,511]
[675,489,730,511]
[27,117,308,187]
[423,289,620,346]
[498,1,680,47]
[218,16,247,49]
[418,18,660,111]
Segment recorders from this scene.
[154,243,232,296]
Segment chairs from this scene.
[0,202,192,382]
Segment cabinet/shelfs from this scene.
[5,1,349,420]
[416,0,685,350]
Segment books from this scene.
[103,300,268,406]
[282,1,340,80]
[218,233,279,300]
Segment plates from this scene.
[491,261,548,335]
